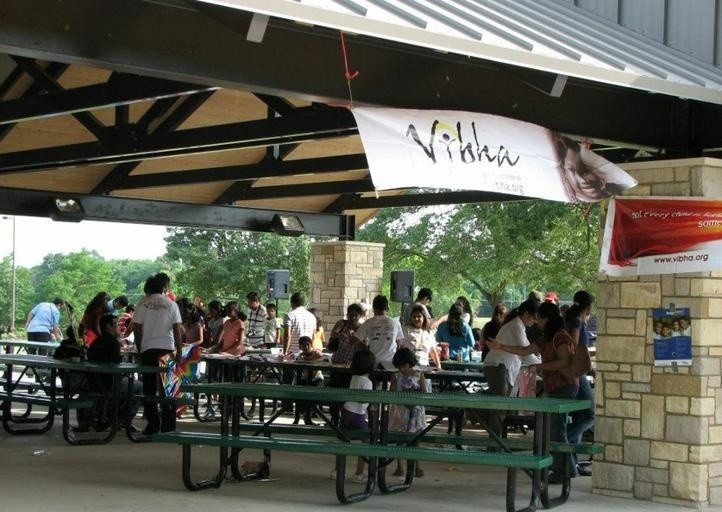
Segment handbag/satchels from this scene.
[325,320,346,352]
[225,462,269,483]
[517,367,537,417]
[552,331,593,381]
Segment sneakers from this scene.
[329,469,349,480]
[393,469,403,476]
[351,472,369,484]
[415,468,424,477]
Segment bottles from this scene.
[457,348,465,364]
[440,342,449,361]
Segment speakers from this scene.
[267,269,289,299]
[390,270,414,303]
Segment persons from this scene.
[552,131,625,204]
[329,348,379,484]
[559,290,596,477]
[25,297,64,355]
[483,301,582,485]
[652,316,690,340]
[389,347,427,478]
[54,273,598,432]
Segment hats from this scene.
[543,292,558,301]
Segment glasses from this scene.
[110,321,119,327]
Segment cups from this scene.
[270,347,283,357]
[418,351,430,365]
[471,350,485,363]
[459,343,470,363]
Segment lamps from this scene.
[47,196,87,223]
[269,213,306,238]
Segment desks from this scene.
[0,341,604,512]
